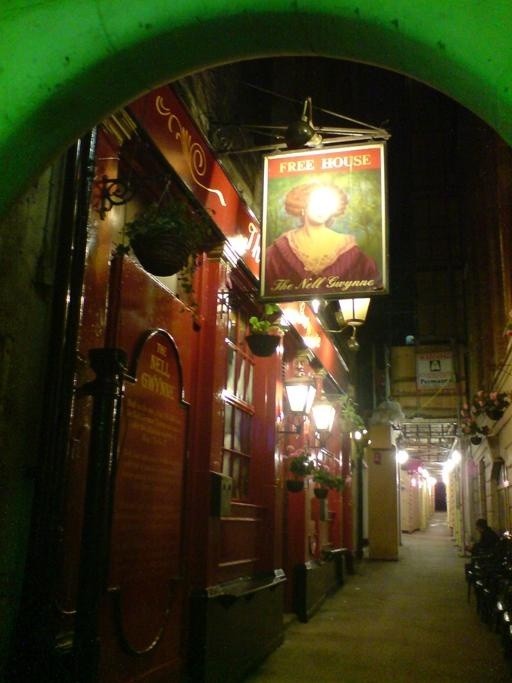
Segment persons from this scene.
[265,181,377,293]
[464,519,499,556]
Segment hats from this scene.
[286,174,348,216]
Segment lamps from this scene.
[307,396,336,448]
[324,297,371,350]
[274,367,318,433]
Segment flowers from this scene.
[471,390,512,413]
[461,403,489,437]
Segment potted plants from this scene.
[312,465,344,498]
[286,453,314,491]
[339,400,364,432]
[245,303,285,356]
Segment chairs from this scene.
[465,538,512,662]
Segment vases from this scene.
[486,409,502,419]
[471,436,481,444]
